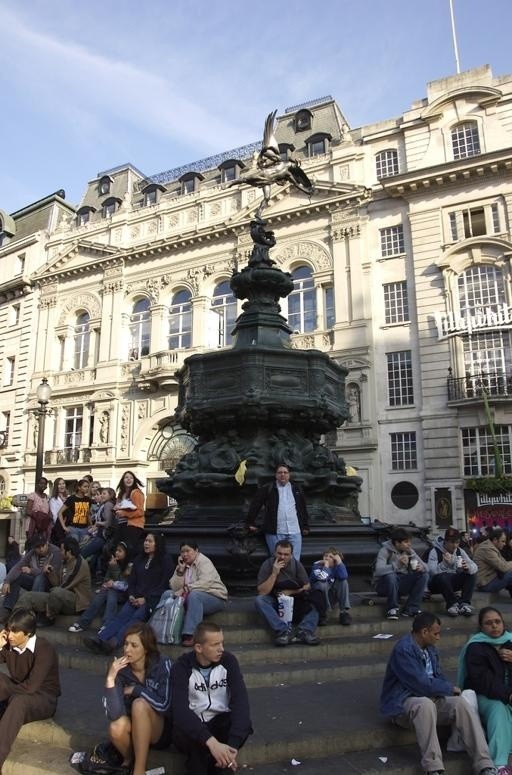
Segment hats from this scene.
[445,529,461,539]
[31,535,47,548]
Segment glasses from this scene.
[276,471,290,474]
[481,620,502,625]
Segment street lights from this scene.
[31,377,52,490]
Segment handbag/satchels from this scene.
[80,741,123,774]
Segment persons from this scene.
[91,512,95,513]
[67,540,136,634]
[244,466,304,563]
[457,530,470,555]
[94,470,145,592]
[23,478,53,546]
[371,528,430,622]
[101,624,168,775]
[0,535,67,623]
[381,613,496,775]
[455,607,512,774]
[257,540,322,645]
[6,537,20,571]
[424,527,481,615]
[479,522,489,536]
[308,547,353,627]
[49,478,71,525]
[493,519,501,531]
[168,621,253,775]
[10,539,94,628]
[503,520,512,540]
[470,528,512,598]
[83,531,177,656]
[89,480,101,500]
[471,522,479,543]
[150,539,230,649]
[84,474,91,486]
[57,478,93,544]
[79,488,117,560]
[89,488,103,522]
[0,609,62,775]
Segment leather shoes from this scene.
[99,638,112,654]
[36,618,56,628]
[83,638,103,655]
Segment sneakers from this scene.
[448,604,459,616]
[498,767,511,775]
[386,608,400,619]
[428,771,445,775]
[339,612,352,624]
[182,635,195,647]
[98,626,106,633]
[69,624,84,632]
[295,630,320,645]
[481,769,497,775]
[402,608,422,617]
[318,616,328,626]
[461,602,476,616]
[274,631,296,646]
